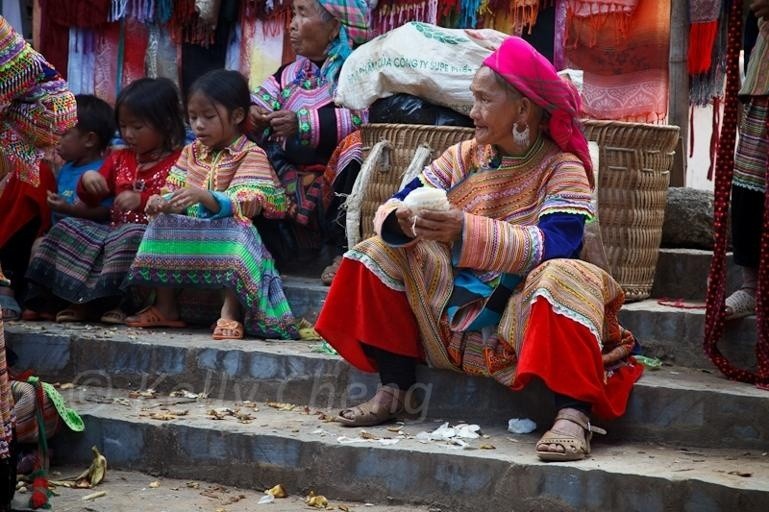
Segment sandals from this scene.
[535,414,607,460]
[336,384,418,427]
[723,283,759,319]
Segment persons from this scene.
[242,1,378,285]
[21,93,115,321]
[23,77,187,325]
[722,0,769,322]
[0,15,78,322]
[117,68,304,341]
[313,37,643,462]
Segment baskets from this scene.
[361,120,681,300]
[360,124,476,241]
[575,121,681,299]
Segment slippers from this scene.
[212,318,244,339]
[321,255,343,284]
[0,294,184,328]
